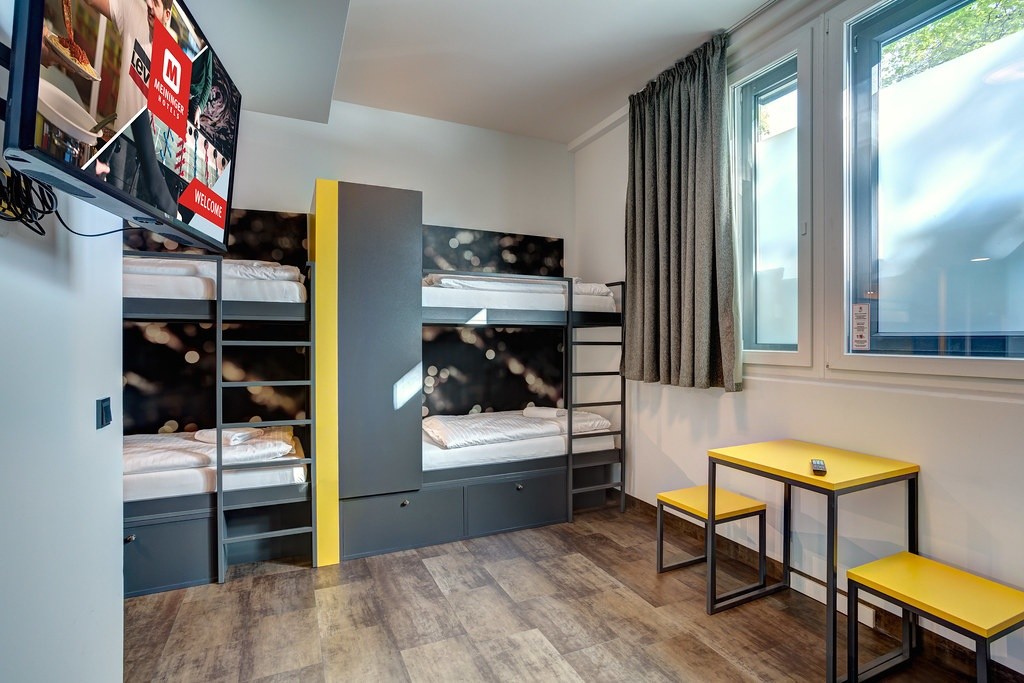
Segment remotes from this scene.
[811,459,827,476]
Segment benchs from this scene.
[846,549,1024,683]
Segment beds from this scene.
[123,250,628,599]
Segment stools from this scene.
[656,483,767,603]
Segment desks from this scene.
[707,437,921,683]
